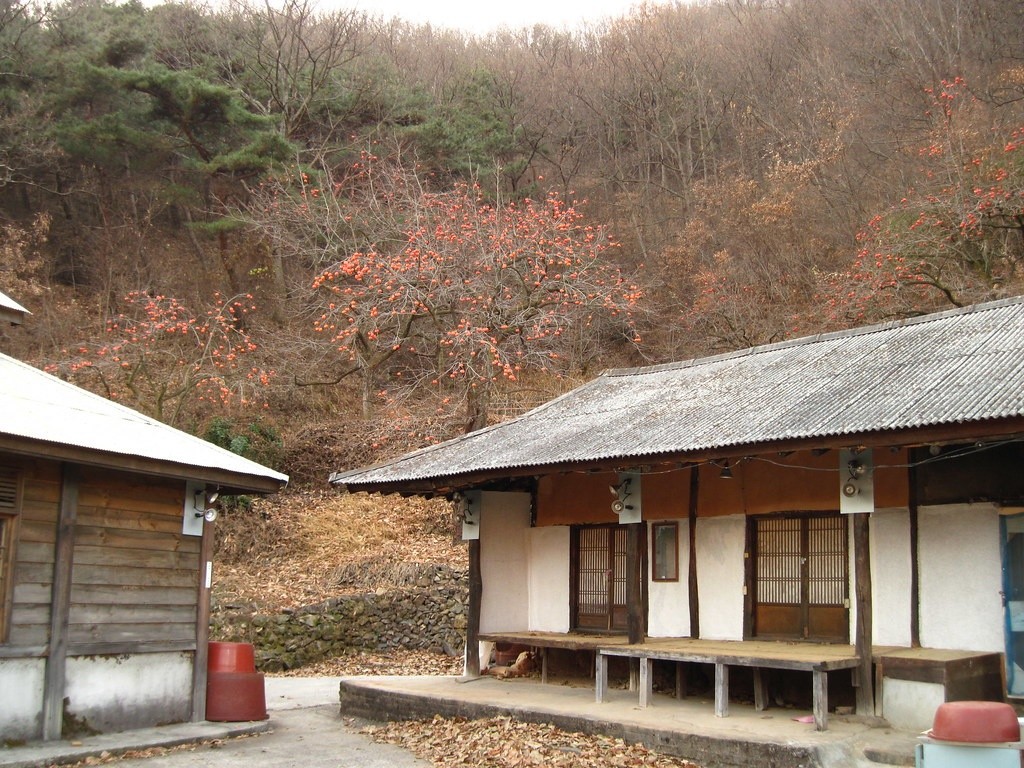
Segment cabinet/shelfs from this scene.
[914,718,1024,768]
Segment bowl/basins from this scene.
[207,672,270,720]
[207,641,255,673]
[928,701,1020,743]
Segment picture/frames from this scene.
[651,520,678,582]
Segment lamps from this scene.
[843,459,867,497]
[719,459,733,478]
[452,491,474,526]
[608,477,634,514]
[193,488,219,522]
